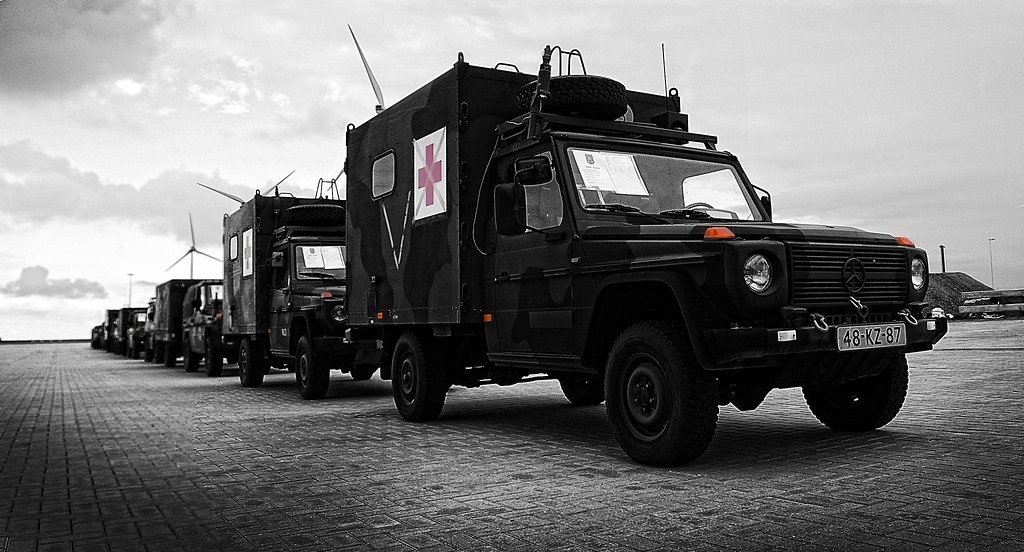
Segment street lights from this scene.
[988,238,996,289]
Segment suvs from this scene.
[220,177,392,401]
[340,43,948,469]
[89,277,239,378]
